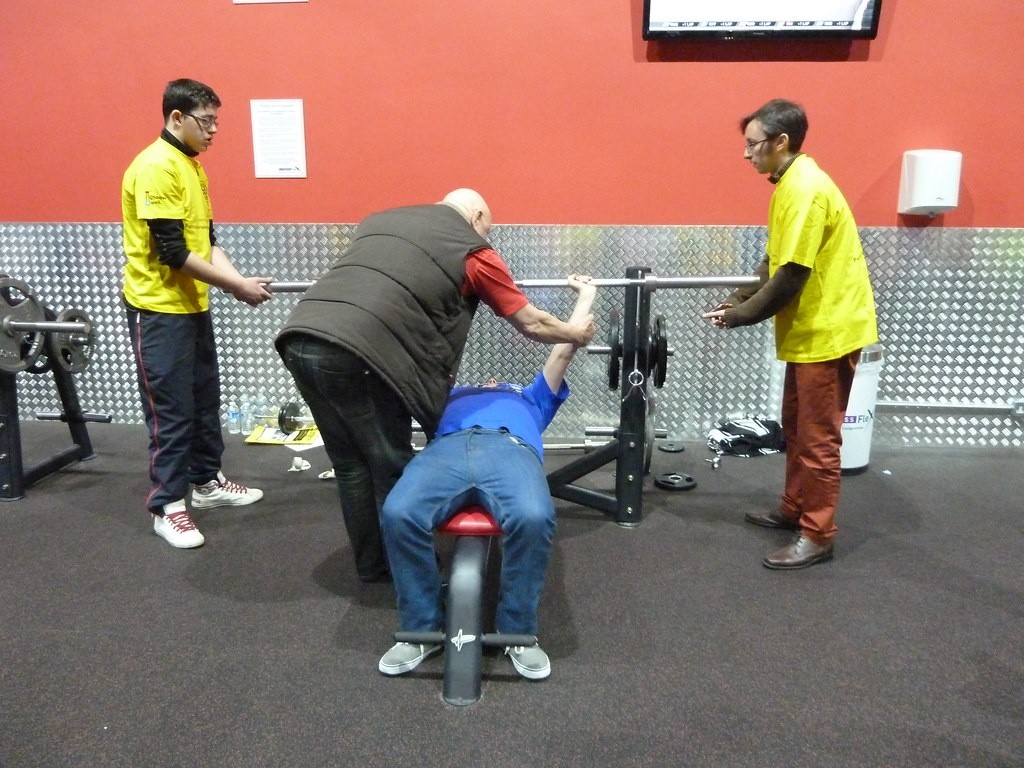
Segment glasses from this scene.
[745,137,777,148]
[180,110,218,127]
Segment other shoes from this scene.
[385,552,440,583]
[361,574,377,581]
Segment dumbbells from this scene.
[254,401,317,436]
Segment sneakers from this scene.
[379,641,442,675]
[191,471,264,510]
[154,498,204,548]
[496,629,551,680]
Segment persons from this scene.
[274,187,597,583]
[376,273,599,681]
[120,77,274,550]
[701,97,880,571]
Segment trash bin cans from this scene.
[838,344,883,477]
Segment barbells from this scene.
[256,271,763,294]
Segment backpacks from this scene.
[706,415,786,470]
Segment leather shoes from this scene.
[745,509,798,530]
[763,534,834,570]
